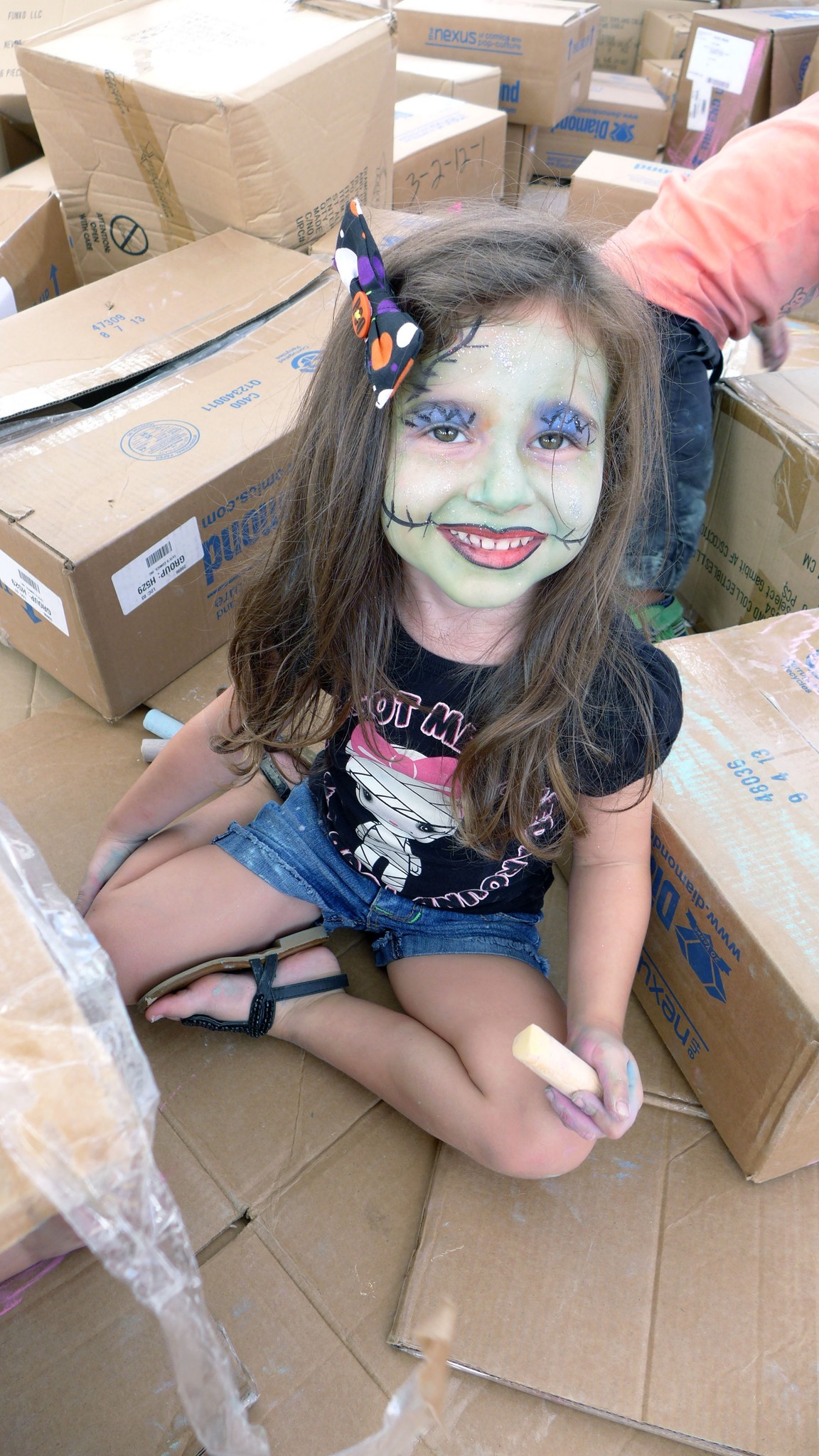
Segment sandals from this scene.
[130,925,351,1038]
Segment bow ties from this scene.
[332,198,424,411]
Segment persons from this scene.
[587,90,819,648]
[67,193,683,1177]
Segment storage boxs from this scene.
[1,1,819,1185]
